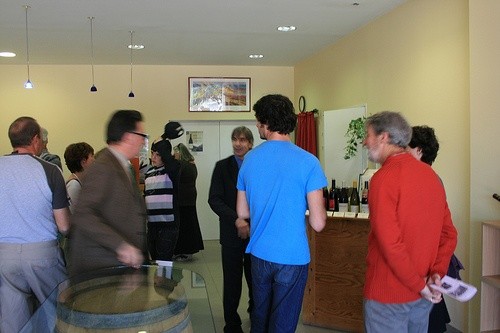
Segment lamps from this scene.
[128,30,134,97]
[25,5,32,88]
[89,17,97,91]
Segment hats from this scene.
[161,122,184,139]
[153,139,172,158]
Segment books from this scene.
[430,275,477,302]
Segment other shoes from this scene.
[154,273,164,286]
[176,254,193,262]
[225,311,242,326]
[164,277,177,290]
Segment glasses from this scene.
[126,131,150,141]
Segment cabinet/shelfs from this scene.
[479,220,500,333]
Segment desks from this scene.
[18,265,216,333]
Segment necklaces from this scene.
[73,173,83,184]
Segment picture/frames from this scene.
[190,270,206,288]
[188,77,251,112]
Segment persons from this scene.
[35,128,64,172]
[63,142,94,215]
[208,126,255,333]
[0,117,70,333]
[363,111,457,333]
[171,143,204,262]
[143,139,192,265]
[406,125,465,333]
[236,94,328,333]
[66,110,147,274]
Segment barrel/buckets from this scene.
[54,273,195,333]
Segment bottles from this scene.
[350,179,360,215]
[338,180,349,212]
[360,180,369,213]
[328,177,339,211]
[323,187,328,211]
